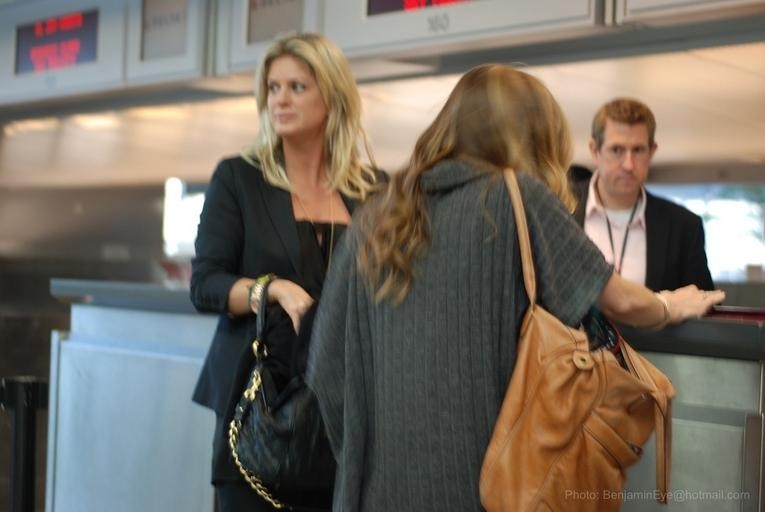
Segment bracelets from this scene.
[646,293,671,332]
[249,273,277,314]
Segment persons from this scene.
[566,98,716,292]
[187,30,391,512]
[303,62,728,512]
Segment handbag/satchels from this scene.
[227,269,327,506]
[476,166,675,508]
